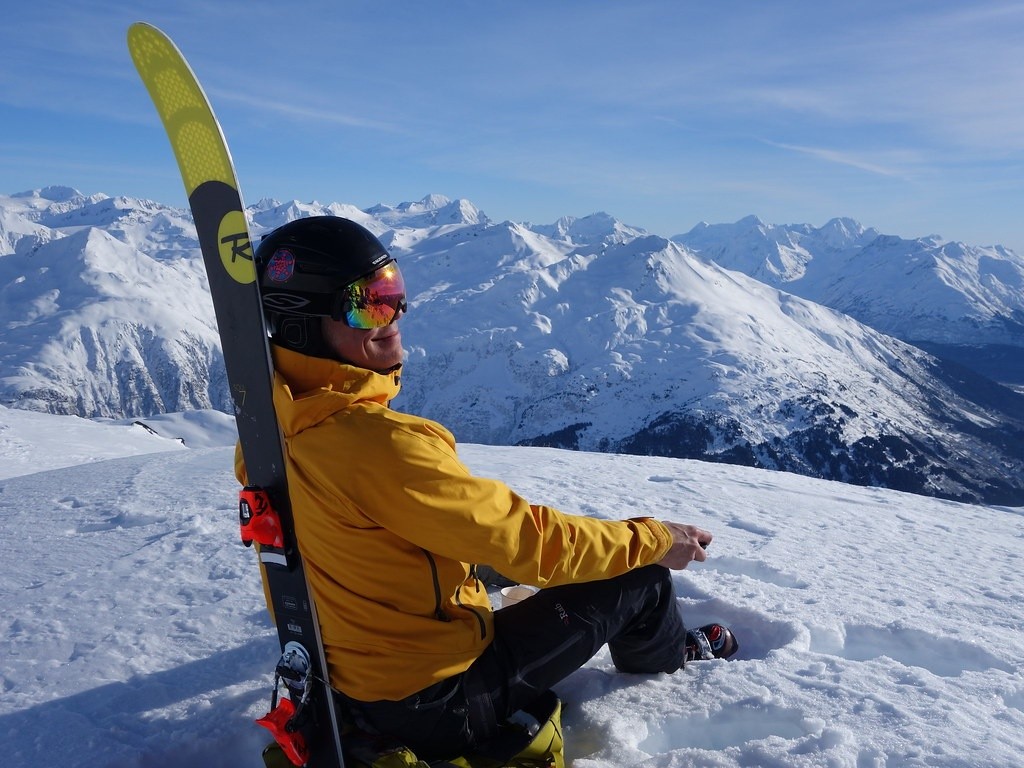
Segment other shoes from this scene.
[683,622,739,660]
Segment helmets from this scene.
[238,215,391,321]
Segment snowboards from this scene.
[124,19,352,768]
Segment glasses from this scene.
[342,258,408,330]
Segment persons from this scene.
[232,215,739,763]
